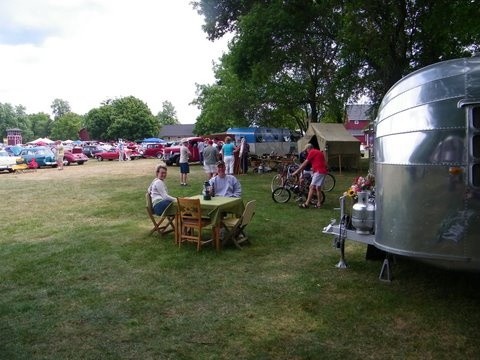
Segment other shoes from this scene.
[181,182,184,184]
[238,233,243,238]
[219,231,224,240]
[184,183,187,185]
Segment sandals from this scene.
[312,205,321,209]
[298,203,309,208]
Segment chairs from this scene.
[145,192,178,240]
[220,200,256,250]
[176,196,215,252]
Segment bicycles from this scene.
[271,157,336,206]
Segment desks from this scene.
[183,194,245,247]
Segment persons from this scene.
[118,141,124,161]
[296,149,316,202]
[203,136,250,178]
[179,139,191,186]
[51,140,64,170]
[292,144,328,208]
[202,161,244,239]
[148,165,177,216]
[198,142,204,165]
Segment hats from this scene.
[216,161,224,167]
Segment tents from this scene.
[144,138,164,142]
[26,138,54,146]
[298,123,360,175]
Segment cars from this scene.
[0,133,236,173]
